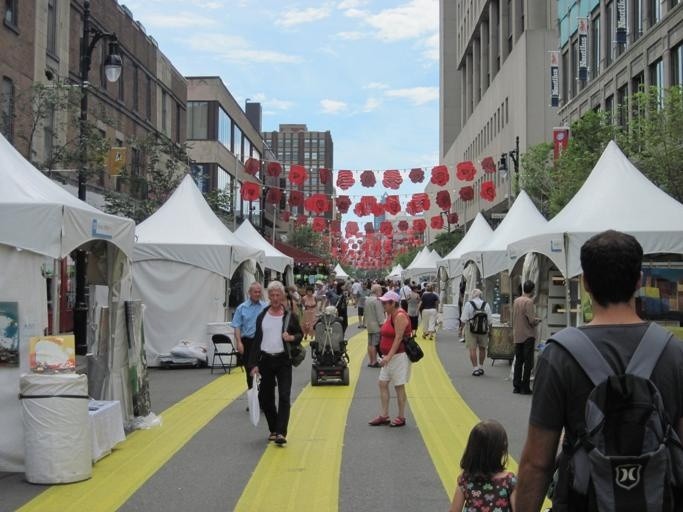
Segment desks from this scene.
[88,400,126,465]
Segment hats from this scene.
[378,291,400,302]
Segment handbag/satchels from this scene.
[402,336,424,363]
[289,344,306,367]
[243,373,261,427]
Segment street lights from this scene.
[72,0,122,355]
[496,136,520,204]
[261,175,286,243]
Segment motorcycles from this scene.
[309,313,349,387]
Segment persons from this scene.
[230,282,268,412]
[368,291,411,427]
[285,276,441,367]
[449,419,517,512]
[514,280,543,394]
[514,229,682,511]
[460,289,493,377]
[246,281,304,444]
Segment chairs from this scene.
[211,334,244,374]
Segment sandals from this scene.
[368,415,390,426]
[390,416,405,427]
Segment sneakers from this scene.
[268,432,287,443]
[473,368,484,376]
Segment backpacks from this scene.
[546,321,683,511]
[469,300,489,335]
